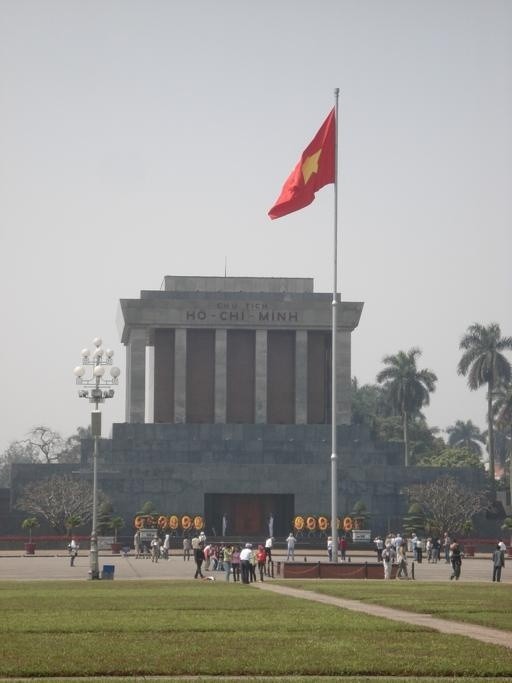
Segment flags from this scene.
[268,105,336,220]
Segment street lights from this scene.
[72,334,123,579]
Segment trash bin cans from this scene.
[101,565,114,580]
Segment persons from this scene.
[328,536,332,562]
[285,532,297,561]
[70,535,80,567]
[491,536,507,584]
[184,530,274,584]
[338,536,349,563]
[408,532,451,565]
[134,530,170,563]
[450,544,464,581]
[375,532,409,583]
